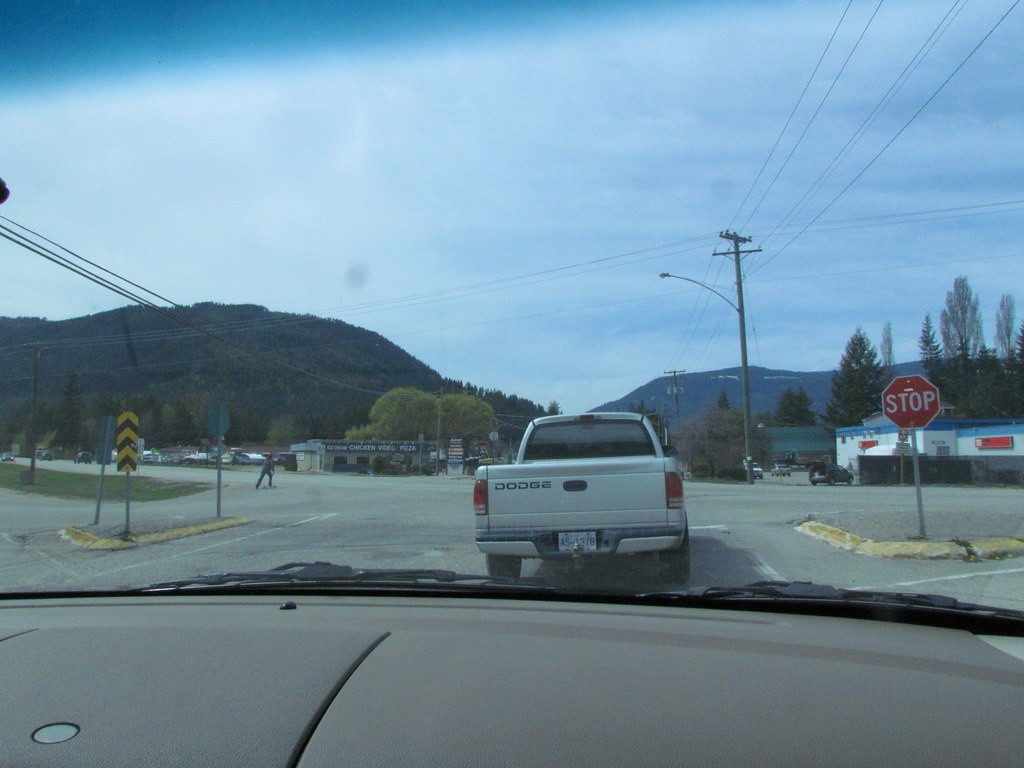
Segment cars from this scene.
[140,450,294,466]
[73,451,92,465]
[771,464,792,477]
[1,453,15,462]
[743,463,763,479]
[40,453,52,461]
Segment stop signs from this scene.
[881,374,940,429]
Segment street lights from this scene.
[659,273,754,484]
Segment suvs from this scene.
[809,464,854,486]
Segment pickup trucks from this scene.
[473,412,690,587]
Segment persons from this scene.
[256,453,275,489]
